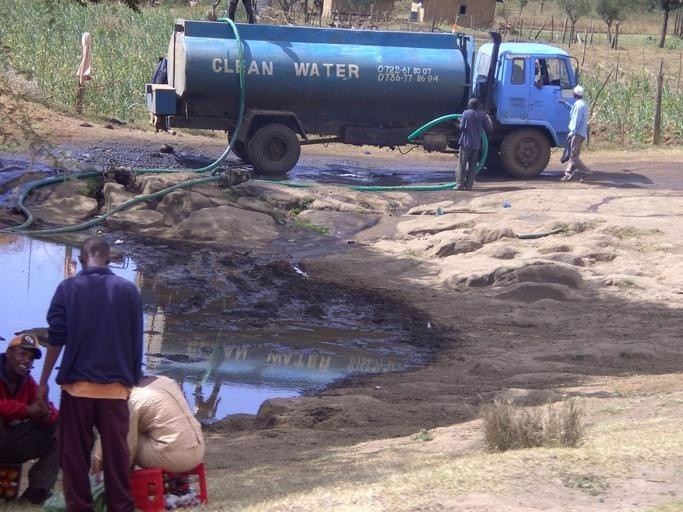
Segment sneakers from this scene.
[452,186,464,190]
[18,487,53,505]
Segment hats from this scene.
[6,333,41,359]
[573,85,584,96]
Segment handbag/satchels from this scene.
[41,469,107,511]
[559,148,569,163]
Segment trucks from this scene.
[144,17,582,180]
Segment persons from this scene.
[532,61,542,87]
[0,334,61,507]
[36,236,144,512]
[557,85,587,184]
[452,98,493,192]
[90,374,205,477]
